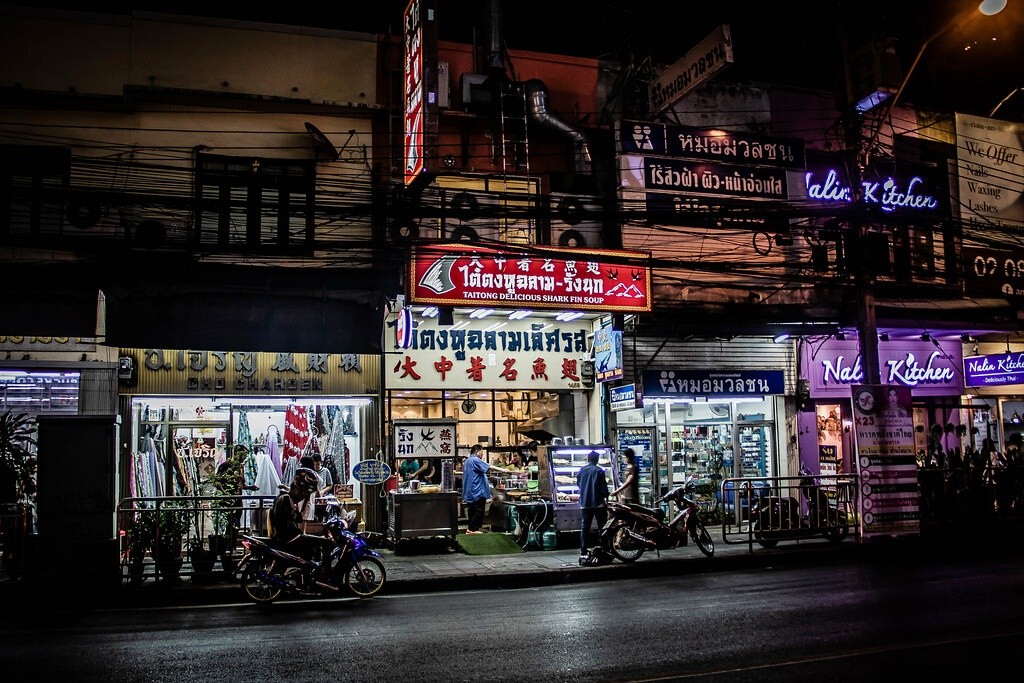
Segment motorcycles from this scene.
[240,495,386,602]
[601,484,717,562]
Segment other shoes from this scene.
[466,530,484,535]
[316,576,339,590]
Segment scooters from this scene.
[740,463,848,548]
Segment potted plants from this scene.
[123,433,266,586]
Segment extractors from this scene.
[515,395,575,441]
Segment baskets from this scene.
[693,497,718,512]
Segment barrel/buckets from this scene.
[527,480,538,491]
[386,475,399,491]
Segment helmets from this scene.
[294,468,321,492]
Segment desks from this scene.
[501,499,554,549]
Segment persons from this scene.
[505,450,526,471]
[610,447,640,548]
[577,452,610,556]
[462,444,511,535]
[213,446,259,539]
[524,440,540,479]
[1006,432,1024,487]
[21,459,37,535]
[884,389,909,417]
[396,458,442,484]
[269,469,341,591]
[312,453,334,496]
[278,456,324,535]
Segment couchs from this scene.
[715,483,771,520]
